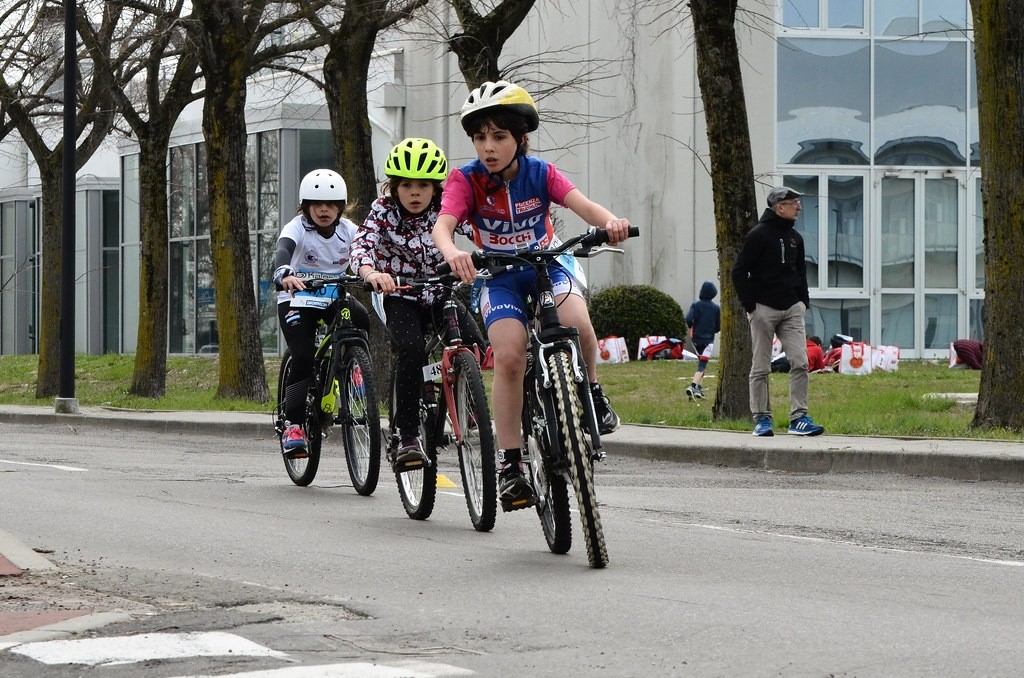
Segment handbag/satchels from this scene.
[596,338,621,364]
[640,340,683,361]
[608,336,629,363]
[637,334,666,359]
[771,357,791,373]
[838,340,900,376]
[811,359,840,374]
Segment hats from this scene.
[767,186,805,207]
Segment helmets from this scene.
[384,137,447,180]
[298,169,347,204]
[460,80,539,133]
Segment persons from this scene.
[733,187,824,437]
[950,340,982,369]
[349,139,486,466]
[271,169,370,451]
[685,282,720,400]
[770,334,846,372]
[432,80,633,501]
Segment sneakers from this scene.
[576,383,620,434]
[694,388,706,399]
[468,414,493,436]
[396,435,422,462]
[787,413,824,435]
[496,460,534,496]
[752,415,774,436]
[686,386,697,401]
[350,368,365,402]
[281,427,310,454]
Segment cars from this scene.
[199,344,219,354]
[262,348,278,357]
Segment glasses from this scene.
[778,199,801,207]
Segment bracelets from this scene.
[364,271,378,283]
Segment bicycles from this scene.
[272,273,381,496]
[435,225,640,570]
[362,273,497,532]
